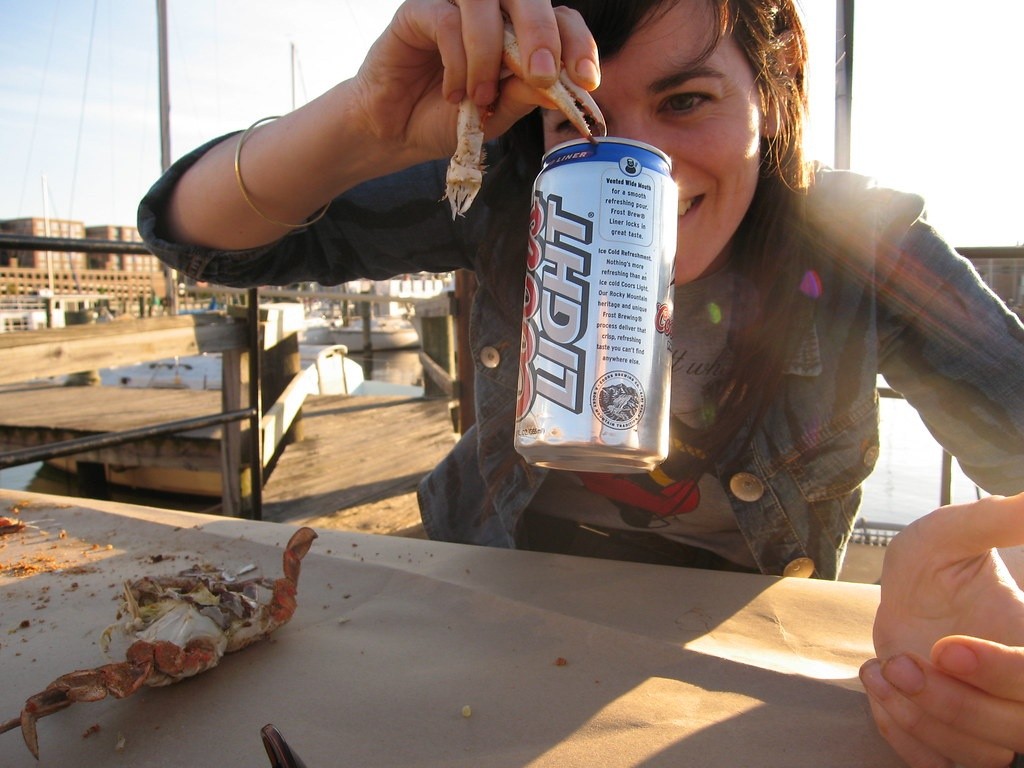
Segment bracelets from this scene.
[234,115,332,228]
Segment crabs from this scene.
[444,24,610,223]
[0,526,319,762]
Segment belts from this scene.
[516,511,760,574]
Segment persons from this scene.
[135,0,1024,768]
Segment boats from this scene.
[332,313,421,353]
[96,340,427,398]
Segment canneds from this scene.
[514,135,680,472]
